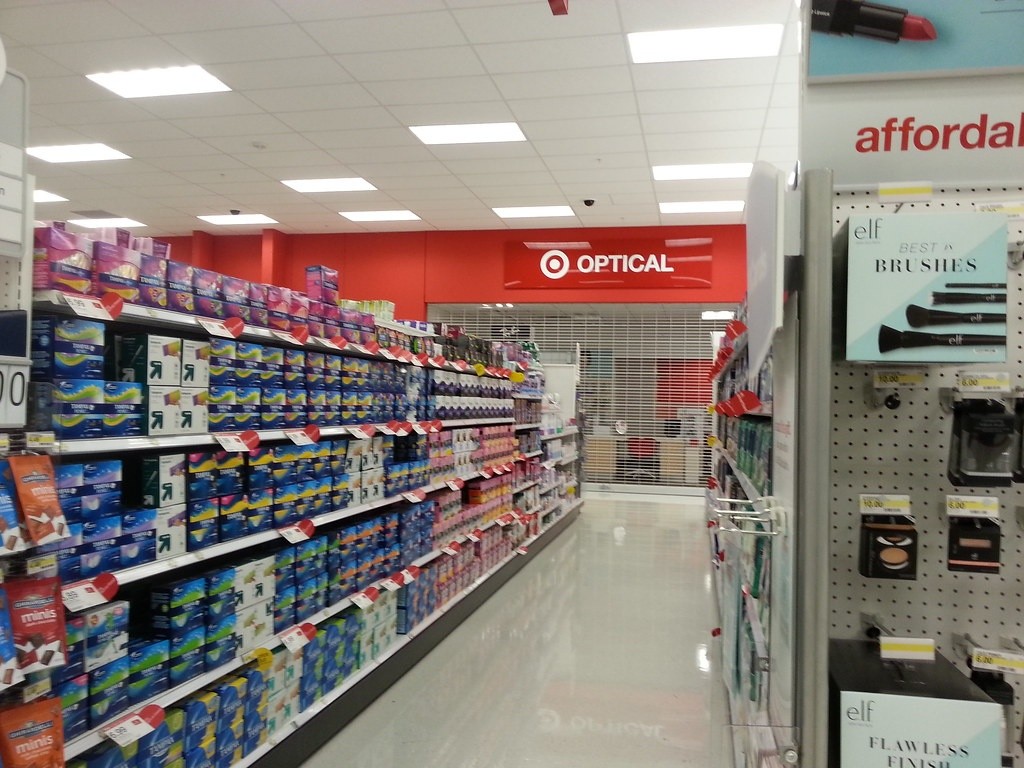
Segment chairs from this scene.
[626,439,657,479]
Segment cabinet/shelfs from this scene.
[0,289,513,768]
[541,423,582,548]
[706,0,1024,768]
[514,365,549,573]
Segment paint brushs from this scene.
[878,282,1006,354]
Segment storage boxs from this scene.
[846,215,1009,364]
[0,218,542,768]
[829,638,1003,768]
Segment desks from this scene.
[583,434,687,483]
